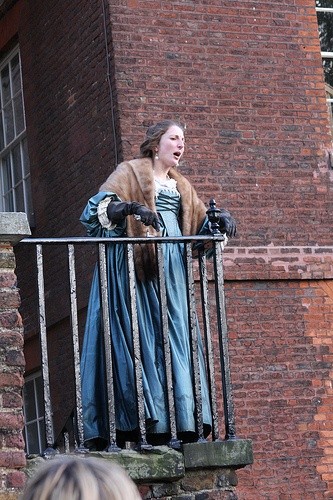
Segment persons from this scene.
[18,457,141,500]
[72,120,237,451]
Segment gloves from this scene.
[97,197,160,232]
[209,208,237,237]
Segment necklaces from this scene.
[152,168,171,188]
